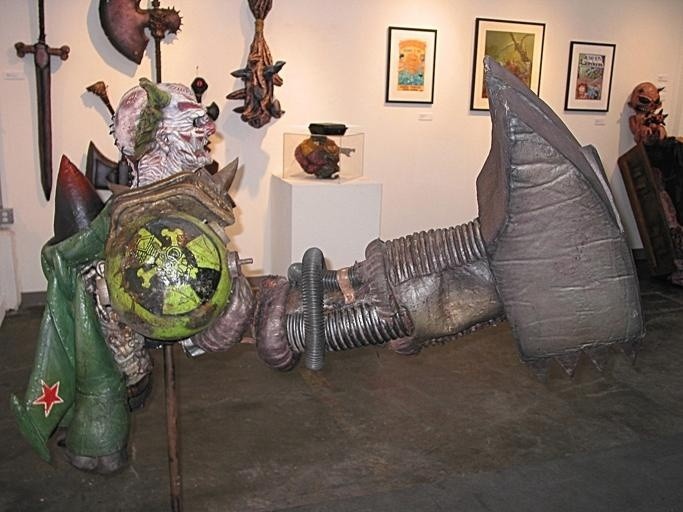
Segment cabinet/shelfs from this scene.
[272,172,388,280]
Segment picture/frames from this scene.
[564,41,616,113]
[386,26,438,106]
[470,17,546,111]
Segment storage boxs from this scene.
[618,138,683,277]
